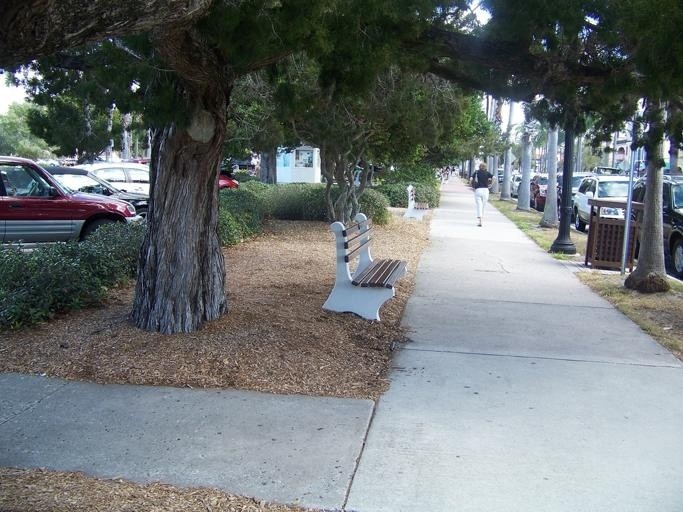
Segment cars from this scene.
[0,152,257,253]
[496,165,683,282]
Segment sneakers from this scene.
[478,225,482,227]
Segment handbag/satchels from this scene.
[472,176,479,188]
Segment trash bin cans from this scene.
[585,198,645,272]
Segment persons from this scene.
[468,161,496,227]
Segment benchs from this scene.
[321,213,408,322]
[404,185,429,221]
[441,174,448,185]
